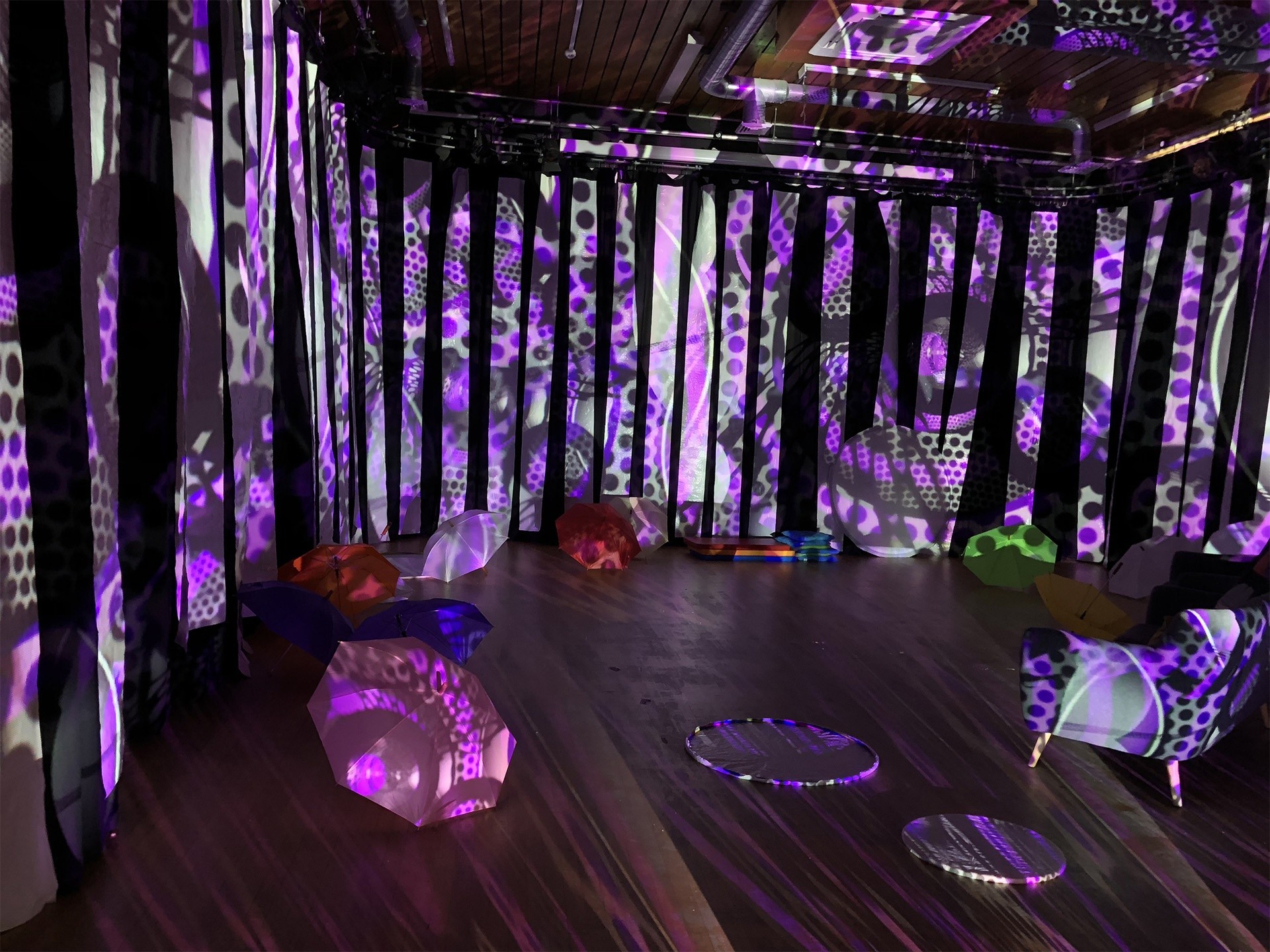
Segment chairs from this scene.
[1019,550,1270,809]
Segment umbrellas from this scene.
[419,507,510,583]
[234,581,354,681]
[1034,559,1133,642]
[964,524,1058,589]
[1107,533,1197,599]
[306,637,519,833]
[611,496,669,553]
[357,598,493,668]
[555,503,642,573]
[279,539,403,625]
